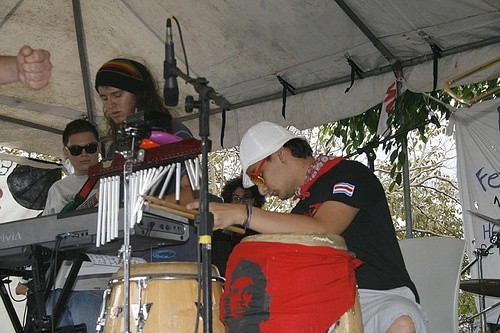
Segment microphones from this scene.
[164,18,179,106]
[426,105,441,128]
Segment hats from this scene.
[239,121,306,188]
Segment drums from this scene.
[220,233,364,333]
[94,261,226,333]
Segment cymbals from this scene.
[459,279,500,298]
[466,209,500,226]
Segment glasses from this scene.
[66,142,99,156]
[249,158,268,186]
[231,194,254,202]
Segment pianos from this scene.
[0,202,190,333]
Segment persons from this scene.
[41,119,116,333]
[95,59,199,262]
[0,46,52,89]
[211,176,265,277]
[186,121,426,333]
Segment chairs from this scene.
[398,237,467,333]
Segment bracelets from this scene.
[243,204,252,229]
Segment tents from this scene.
[0,0,500,239]
